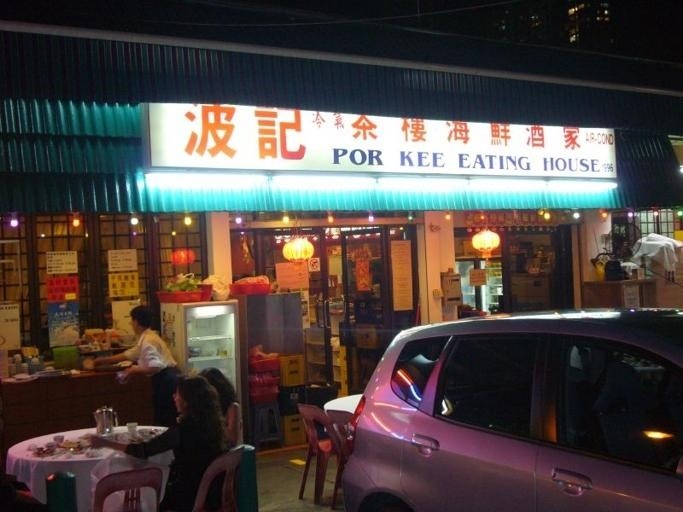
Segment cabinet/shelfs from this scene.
[583,279,657,308]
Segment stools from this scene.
[247,399,284,452]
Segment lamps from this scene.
[10,214,19,228]
[282,237,315,265]
[470,226,501,259]
[130,213,139,225]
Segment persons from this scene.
[94,304,186,428]
[79,375,226,512]
[198,366,244,448]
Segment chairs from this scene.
[296,402,357,510]
[191,446,244,512]
[94,467,163,512]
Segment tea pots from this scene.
[93,406,119,433]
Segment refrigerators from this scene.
[162,301,241,404]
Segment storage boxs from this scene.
[279,386,306,415]
[278,352,306,387]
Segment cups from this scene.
[128,423,137,432]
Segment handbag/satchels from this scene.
[89,449,170,511]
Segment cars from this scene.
[341,308,682,512]
